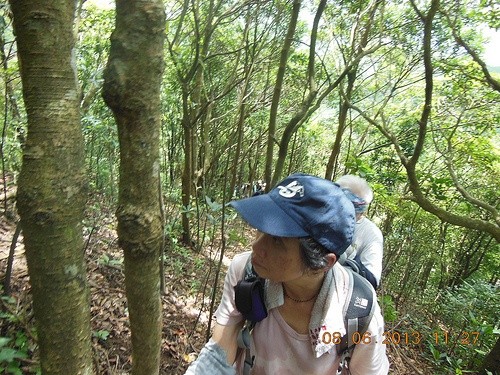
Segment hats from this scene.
[232,174,356,256]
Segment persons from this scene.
[184,173,389,375]
[333,174,384,288]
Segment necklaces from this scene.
[284,291,318,302]
